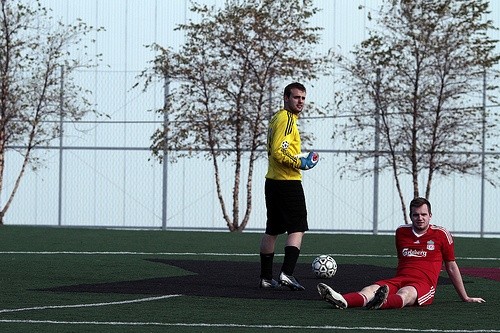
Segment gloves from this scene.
[298,152,320,170]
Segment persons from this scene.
[259,82,319,290]
[317,197,487,310]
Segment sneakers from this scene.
[365,284,389,310]
[316,283,348,310]
[258,278,286,289]
[278,271,306,290]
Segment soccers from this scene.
[312,255,337,277]
